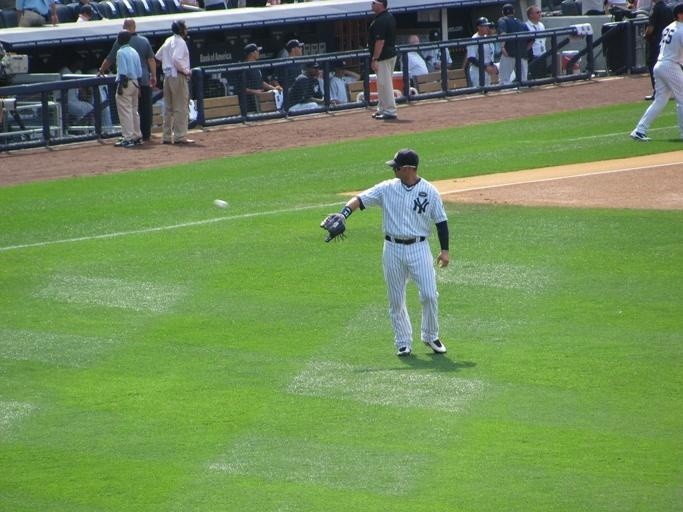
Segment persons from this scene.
[321,149,452,358]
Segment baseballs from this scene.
[212,199,227,208]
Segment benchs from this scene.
[152,68,467,127]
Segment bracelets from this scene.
[341,206,352,217]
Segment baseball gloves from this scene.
[320,213,347,242]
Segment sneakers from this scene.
[424,339,446,353]
[631,130,651,141]
[372,112,397,119]
[396,347,410,356]
[114,138,144,148]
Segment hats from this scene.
[286,40,305,50]
[244,43,263,53]
[429,30,439,40]
[477,17,491,27]
[386,149,417,169]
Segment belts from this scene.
[385,235,425,245]
